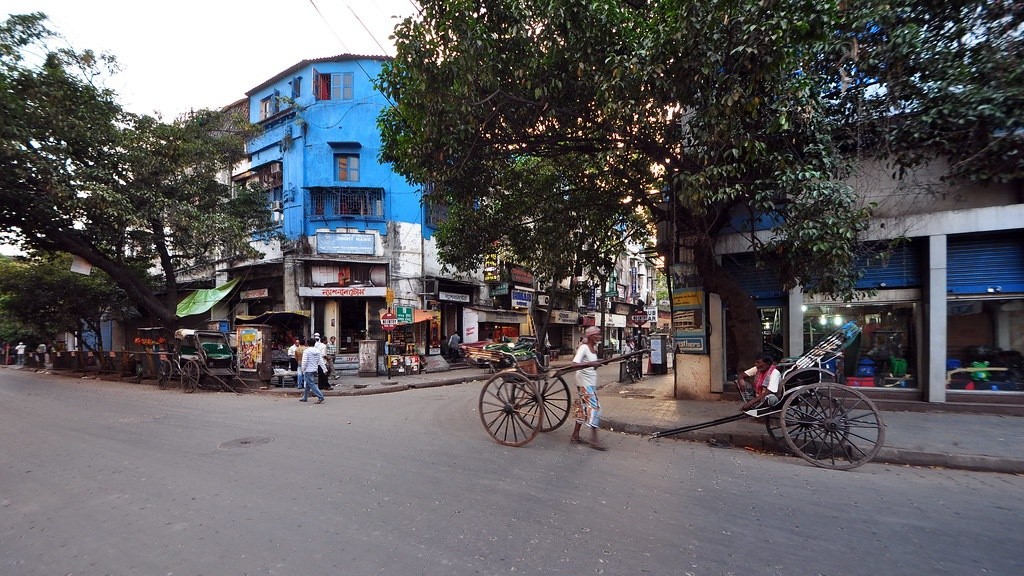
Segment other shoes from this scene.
[571,436,587,444]
[314,399,324,404]
[299,398,307,402]
[335,375,340,380]
[328,377,331,379]
[586,440,608,450]
[325,387,333,390]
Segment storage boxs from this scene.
[845,376,875,387]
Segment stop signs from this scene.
[380,313,397,331]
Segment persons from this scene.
[15,342,25,365]
[439,331,460,363]
[738,355,783,411]
[571,327,606,450]
[288,333,336,403]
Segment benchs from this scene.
[201,342,232,359]
[180,345,199,361]
[504,352,546,377]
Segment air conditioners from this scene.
[273,200,281,210]
[271,163,283,174]
[537,295,550,305]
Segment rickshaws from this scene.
[157,328,253,395]
[460,340,656,446]
[648,322,885,469]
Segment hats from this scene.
[585,326,601,338]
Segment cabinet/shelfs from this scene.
[387,323,421,380]
